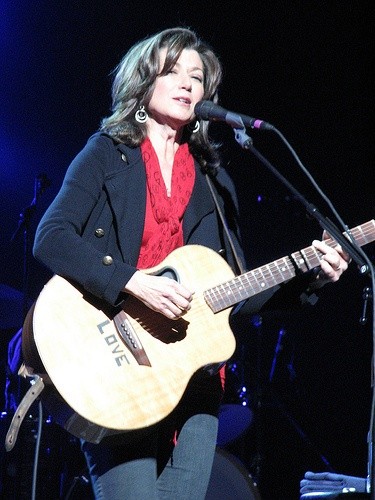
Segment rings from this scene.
[334,260,343,272]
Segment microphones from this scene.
[194,99,274,131]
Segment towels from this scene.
[298,470,369,499]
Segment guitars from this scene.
[22,219,375,445]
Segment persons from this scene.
[32,28,356,500]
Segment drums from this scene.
[68,447,260,500]
[216,362,257,446]
